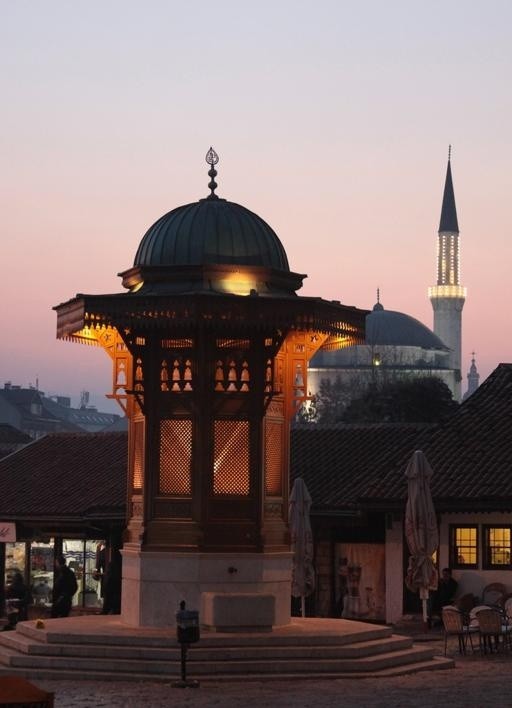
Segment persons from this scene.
[1,573,35,632]
[51,555,79,618]
[431,568,458,619]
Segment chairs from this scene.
[441,583,512,657]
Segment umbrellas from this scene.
[289,475,319,617]
[403,448,441,635]
